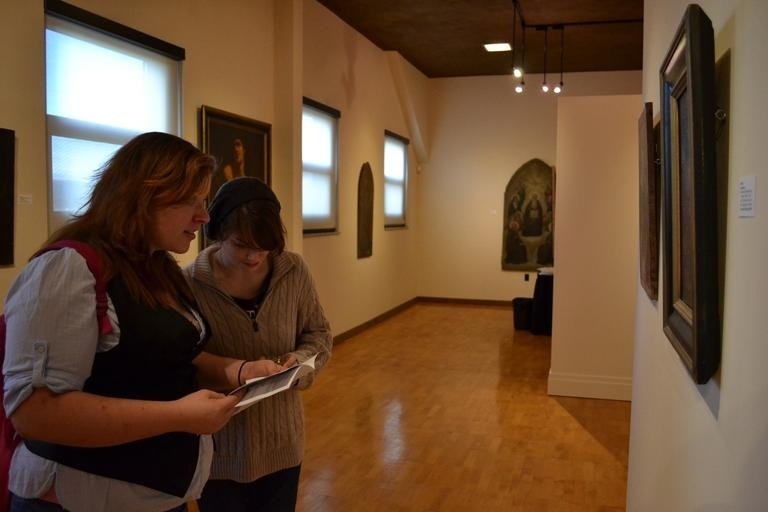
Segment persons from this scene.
[180,175,332,511]
[220,135,262,186]
[508,182,553,266]
[0,131,291,510]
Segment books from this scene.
[226,350,319,417]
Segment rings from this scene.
[274,364,282,371]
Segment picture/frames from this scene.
[656,1,726,386]
[636,101,659,303]
[198,103,273,256]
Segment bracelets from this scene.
[237,360,248,387]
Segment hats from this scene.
[203,177,281,240]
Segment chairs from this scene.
[509,272,554,336]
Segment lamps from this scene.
[509,1,569,95]
